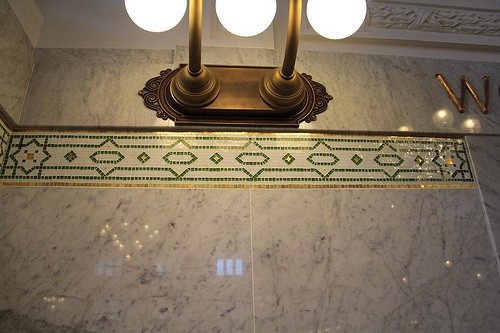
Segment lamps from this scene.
[124,0,367,132]
